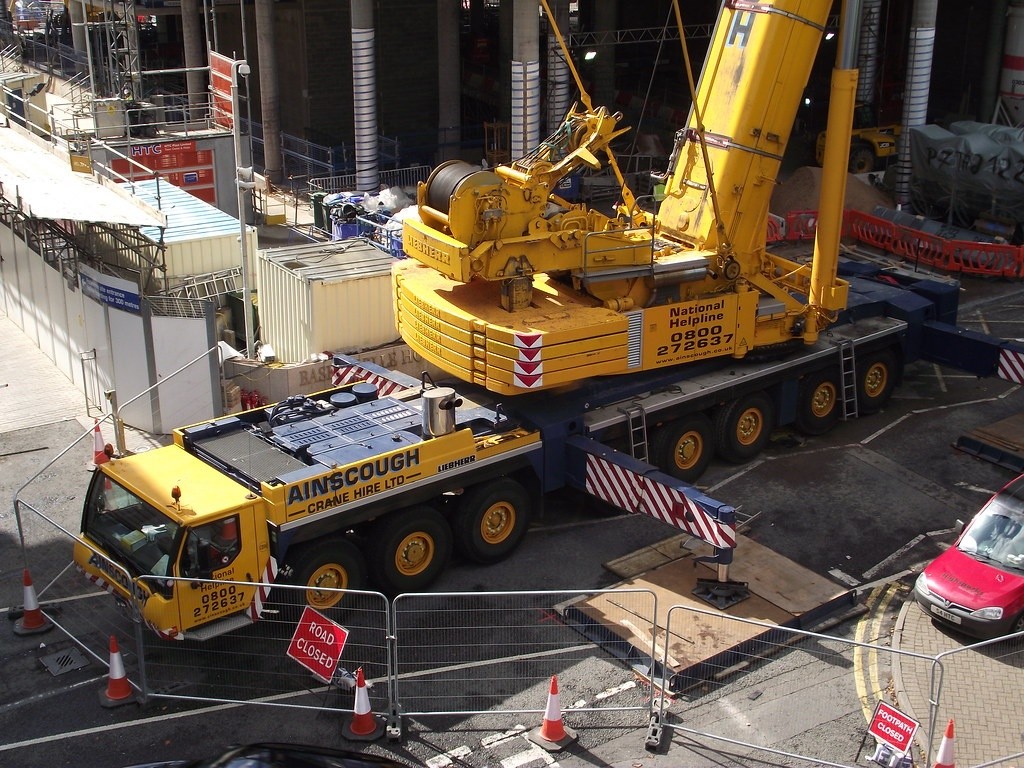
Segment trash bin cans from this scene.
[307,193,327,235]
[321,192,344,237]
[343,190,406,260]
[329,215,360,241]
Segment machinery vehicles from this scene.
[76,0,1024,644]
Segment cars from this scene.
[914,474,1024,645]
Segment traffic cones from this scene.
[97,635,140,708]
[13,569,55,636]
[933,718,955,768]
[527,675,579,753]
[341,668,386,742]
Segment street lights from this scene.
[230,60,256,361]
